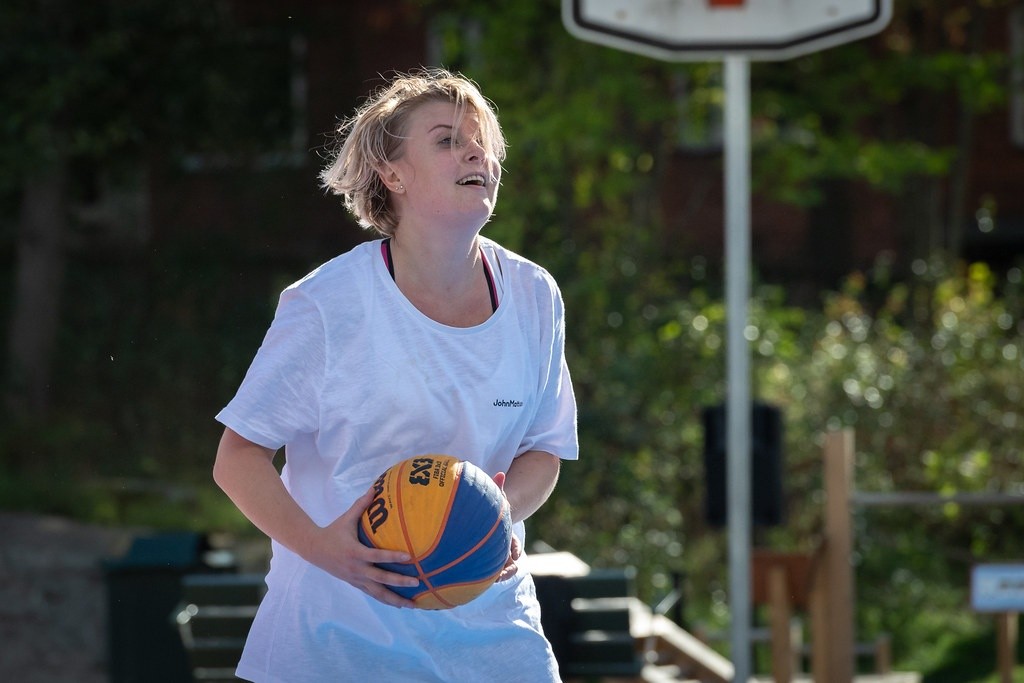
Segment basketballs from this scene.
[357,454,513,611]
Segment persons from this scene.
[212,74,576,683]
